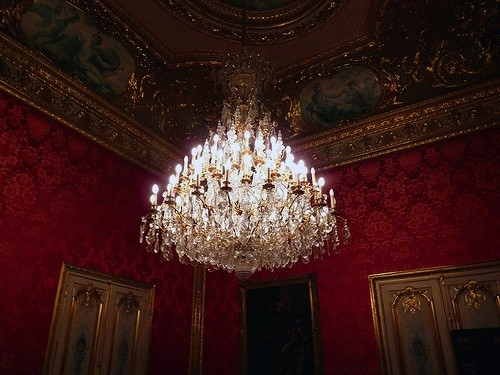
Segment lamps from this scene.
[138,0,351,281]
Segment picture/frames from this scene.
[40,261,157,375]
[367,260,500,375]
[238,272,324,375]
